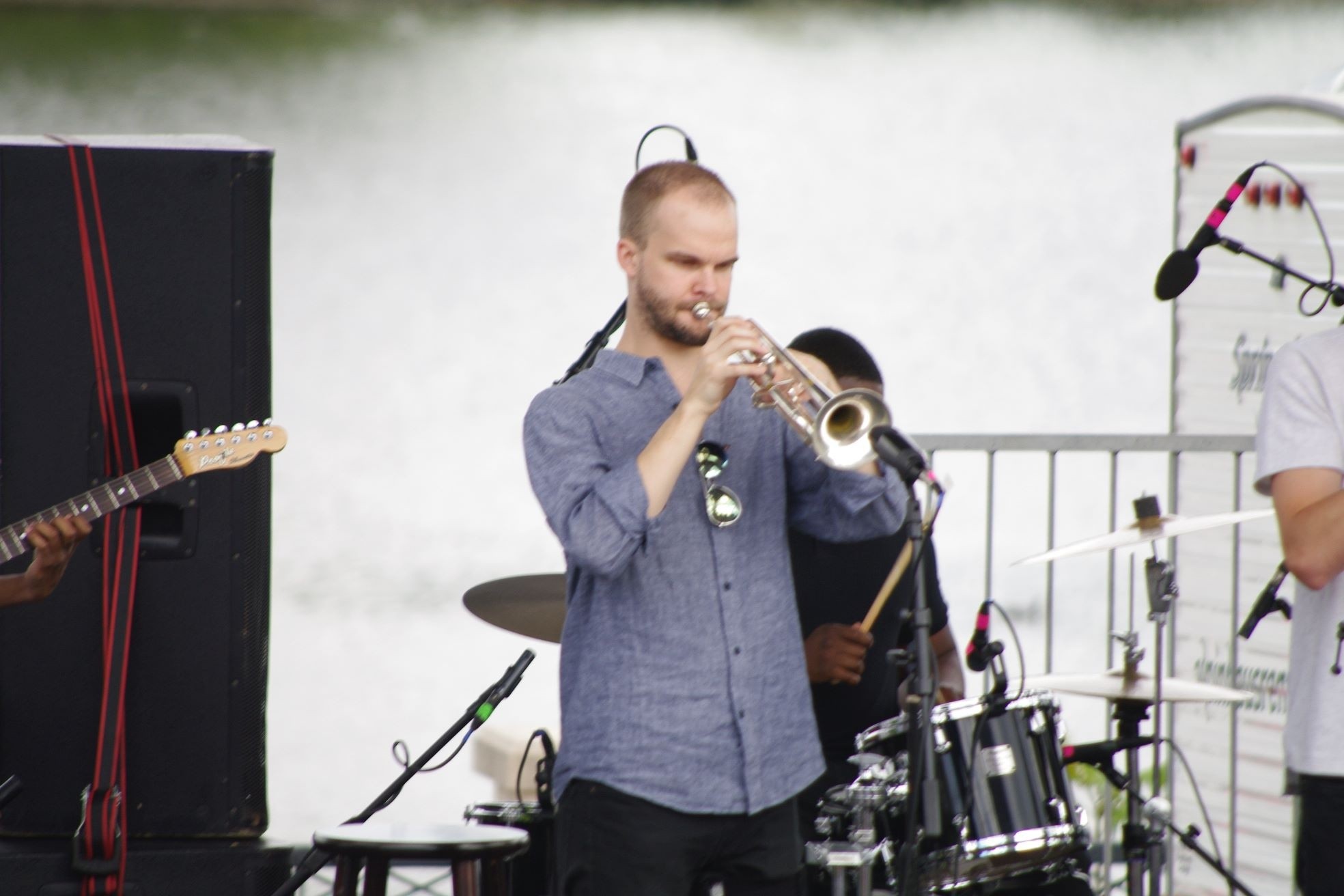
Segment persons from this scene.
[0,514,93,605]
[521,161,906,896]
[1255,322,1344,894]
[779,327,968,841]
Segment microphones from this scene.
[1062,736,1155,765]
[868,425,942,496]
[965,602,991,672]
[472,650,535,730]
[1155,167,1256,300]
[540,733,559,771]
[1239,561,1291,640]
[685,138,699,163]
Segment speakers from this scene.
[0,144,273,839]
[0,840,296,896]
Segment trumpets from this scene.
[690,301,894,472]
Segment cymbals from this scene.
[1007,668,1258,702]
[462,573,569,646]
[1008,506,1277,568]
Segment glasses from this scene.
[694,438,742,528]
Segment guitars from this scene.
[0,417,287,567]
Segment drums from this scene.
[818,782,903,876]
[853,686,1094,895]
[450,801,543,896]
